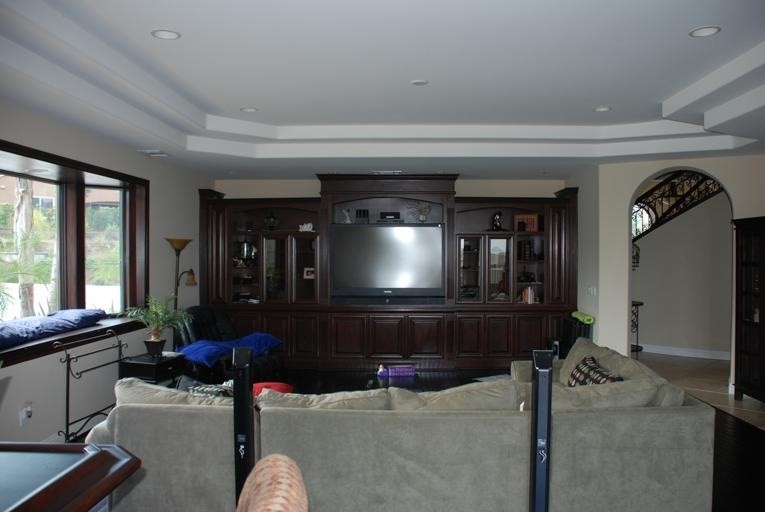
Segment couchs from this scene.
[84,336,716,511]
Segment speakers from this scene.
[533,349,550,512]
[234,349,254,507]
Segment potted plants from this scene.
[114,290,195,356]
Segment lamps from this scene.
[163,237,198,383]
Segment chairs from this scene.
[178,303,273,384]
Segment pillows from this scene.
[568,354,624,387]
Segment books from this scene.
[517,285,541,304]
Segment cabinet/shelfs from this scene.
[730,216,765,402]
[118,351,186,388]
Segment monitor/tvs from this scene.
[328,224,445,308]
